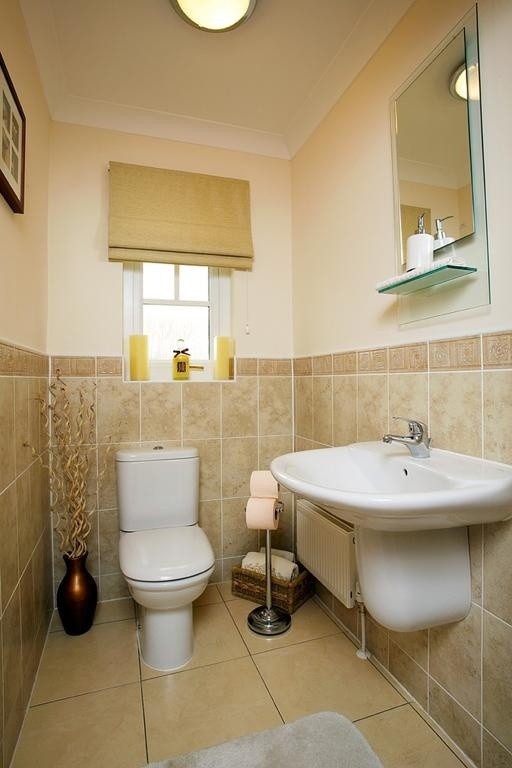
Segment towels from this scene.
[243,547,298,581]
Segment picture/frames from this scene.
[0,53,26,215]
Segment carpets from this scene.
[148,710,384,768]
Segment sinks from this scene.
[270,440,511,531]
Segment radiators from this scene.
[292,504,359,612]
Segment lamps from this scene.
[172,0,260,32]
[446,60,470,104]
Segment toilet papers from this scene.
[246,496,280,530]
[249,470,281,498]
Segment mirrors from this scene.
[379,29,493,266]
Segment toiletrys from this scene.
[434,216,454,249]
[406,214,434,272]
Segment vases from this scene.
[55,549,99,638]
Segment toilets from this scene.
[114,446,215,671]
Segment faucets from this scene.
[383,417,430,458]
